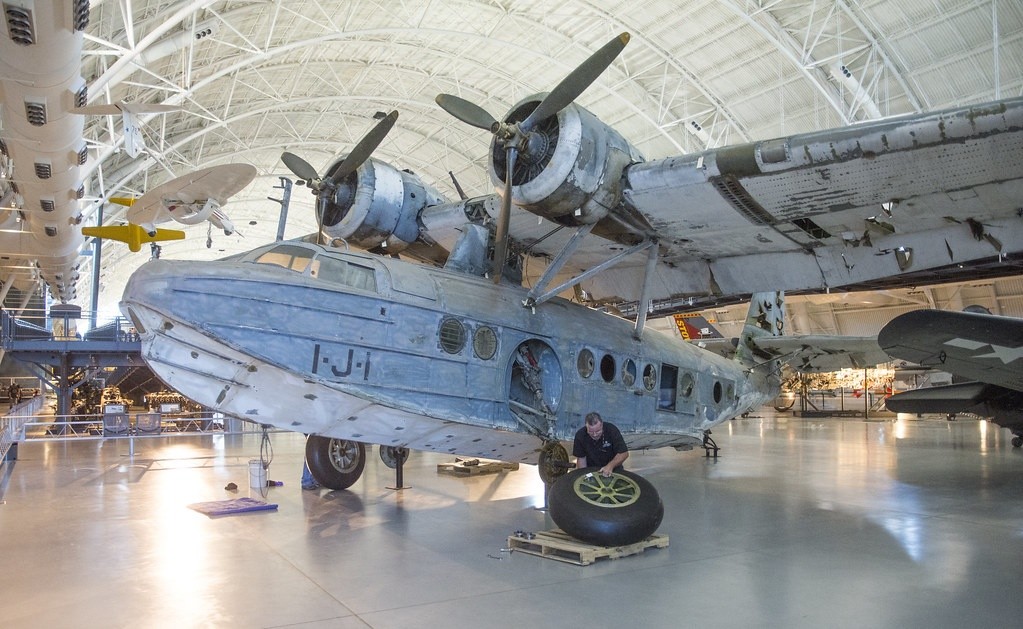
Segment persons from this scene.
[2,383,22,409]
[572,412,629,477]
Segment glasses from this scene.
[587,425,603,435]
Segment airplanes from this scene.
[877,304,1023,448]
[119,30,1023,487]
